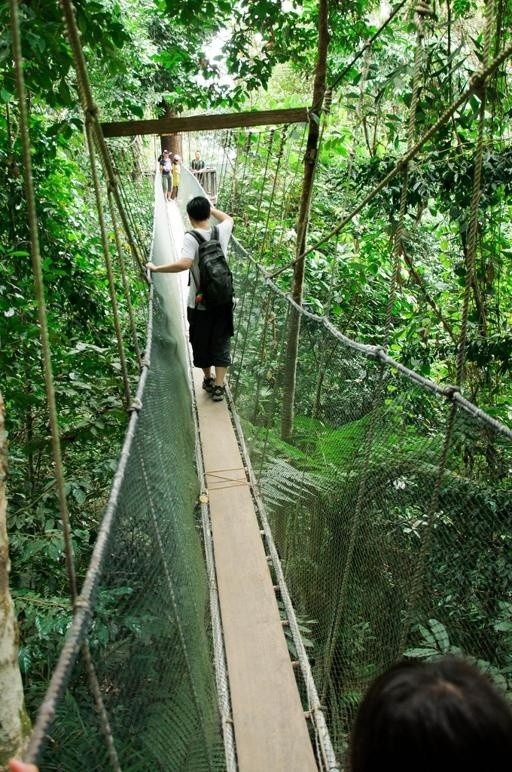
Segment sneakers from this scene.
[202,372,226,401]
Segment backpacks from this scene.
[185,226,234,315]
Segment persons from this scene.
[191,150,204,191]
[9,657,511,772]
[145,196,236,402]
[157,149,183,202]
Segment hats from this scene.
[174,155,181,160]
